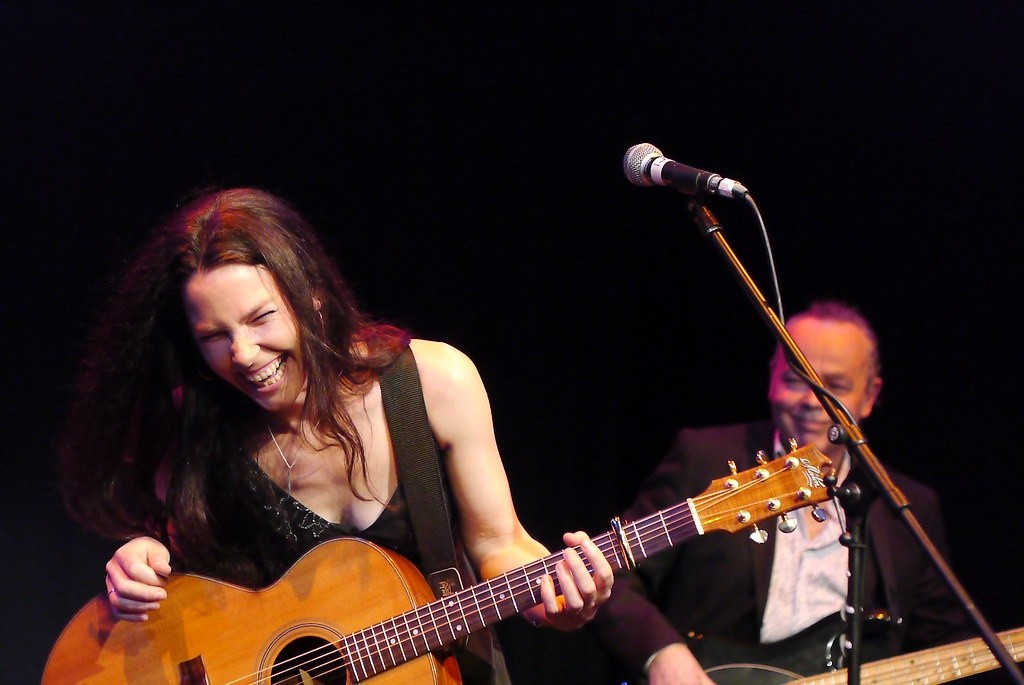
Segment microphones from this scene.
[624,143,749,198]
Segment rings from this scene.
[107,588,116,596]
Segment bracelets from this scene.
[644,650,659,676]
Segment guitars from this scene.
[39,436,864,685]
[704,626,1024,685]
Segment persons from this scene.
[601,296,987,685]
[54,183,615,685]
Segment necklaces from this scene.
[264,420,320,500]
